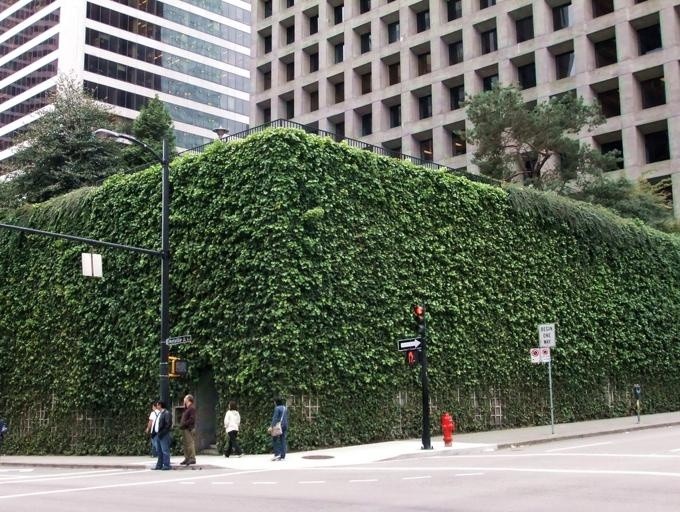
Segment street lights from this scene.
[95,126,169,407]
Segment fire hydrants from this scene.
[440,412,454,448]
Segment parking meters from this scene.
[633,384,641,422]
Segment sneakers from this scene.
[151,466,171,470]
[271,455,284,461]
[222,453,244,458]
[180,458,196,465]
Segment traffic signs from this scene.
[398,339,425,351]
[539,324,557,348]
[166,334,191,347]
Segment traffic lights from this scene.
[173,360,187,374]
[406,350,418,368]
[412,303,427,332]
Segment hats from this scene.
[212,125,230,134]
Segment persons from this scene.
[178,393,197,465]
[268,398,289,461]
[145,404,161,458]
[223,400,245,458]
[150,401,172,470]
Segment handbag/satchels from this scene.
[271,421,282,437]
